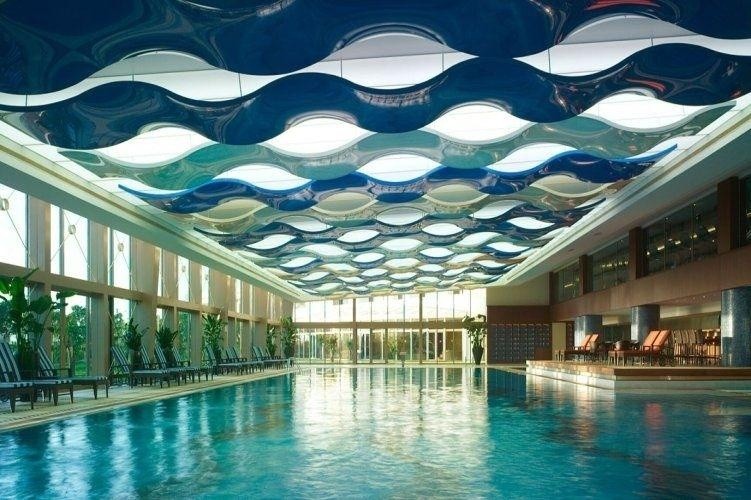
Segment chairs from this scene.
[0,334,292,412]
[554,327,722,368]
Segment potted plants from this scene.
[462,313,487,364]
[153,325,180,368]
[121,317,150,371]
[0,267,76,401]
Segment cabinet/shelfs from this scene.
[488,322,551,361]
